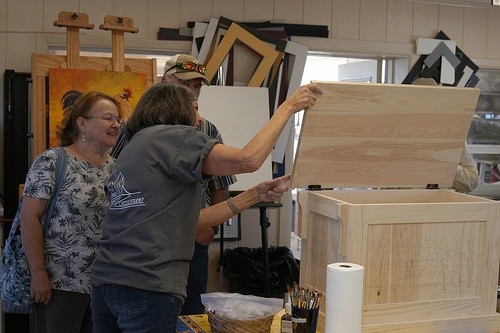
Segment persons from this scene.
[21,91,126,333]
[89,83,322,333]
[107,54,239,317]
[372,78,479,194]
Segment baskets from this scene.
[206,307,273,333]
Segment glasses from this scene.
[85,113,124,126]
[161,62,207,77]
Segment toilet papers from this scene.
[324,262,364,333]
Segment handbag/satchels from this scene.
[0,145,67,313]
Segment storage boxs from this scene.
[288,78,500,333]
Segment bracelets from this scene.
[227,197,239,215]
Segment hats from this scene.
[164,54,209,86]
[413,78,437,86]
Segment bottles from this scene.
[281,303,291,333]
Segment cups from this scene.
[291,305,319,333]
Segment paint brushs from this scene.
[286,283,324,309]
